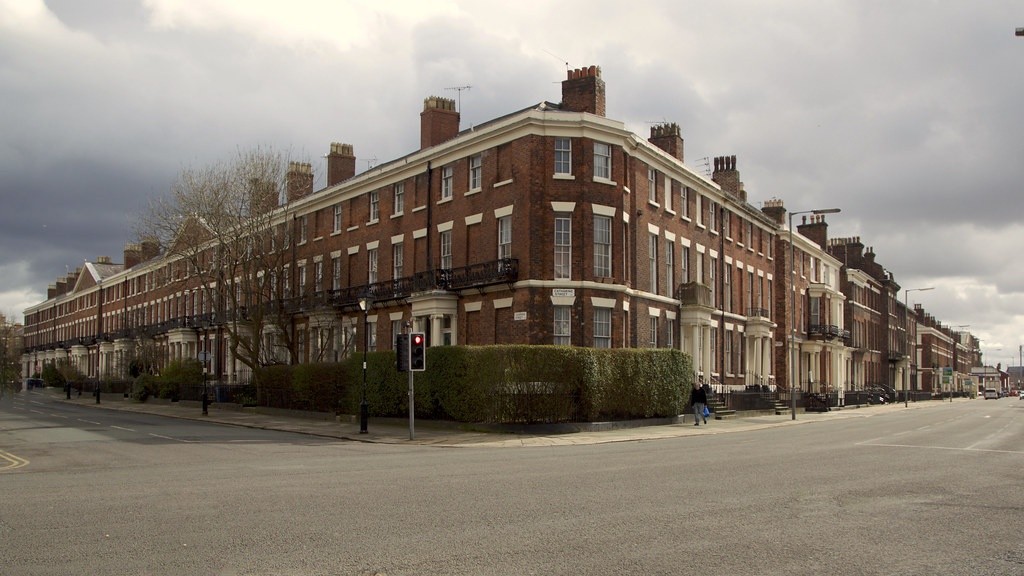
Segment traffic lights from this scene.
[409,332,425,371]
[395,333,409,372]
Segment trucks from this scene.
[985,380,1002,398]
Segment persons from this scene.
[691,382,708,426]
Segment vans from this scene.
[985,388,998,399]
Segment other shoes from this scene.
[704,419,706,424]
[694,423,699,426]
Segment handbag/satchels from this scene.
[704,407,709,417]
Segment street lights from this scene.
[201,314,210,416]
[95,334,102,404]
[356,287,375,434]
[789,209,841,420]
[950,325,970,402]
[905,288,935,408]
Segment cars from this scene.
[1002,389,1024,400]
